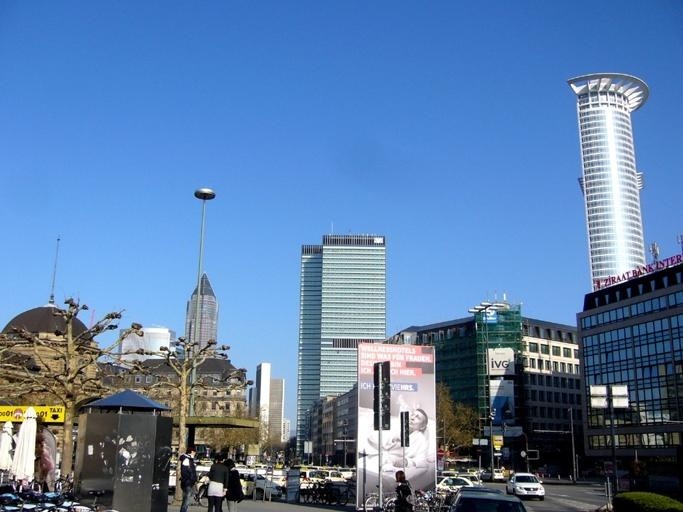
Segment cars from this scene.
[449,487,527,512]
[438,476,473,492]
[166,452,357,499]
[460,475,483,487]
[439,469,459,478]
[505,473,546,500]
[481,468,505,481]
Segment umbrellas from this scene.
[10,406,37,492]
[0,420,14,483]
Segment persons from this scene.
[365,408,430,475]
[223,459,241,512]
[179,446,197,511]
[206,454,229,512]
[392,470,413,512]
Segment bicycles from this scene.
[0,475,121,511]
[363,483,446,510]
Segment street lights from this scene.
[468,301,511,412]
[186,188,215,417]
[487,412,496,483]
[566,405,590,485]
